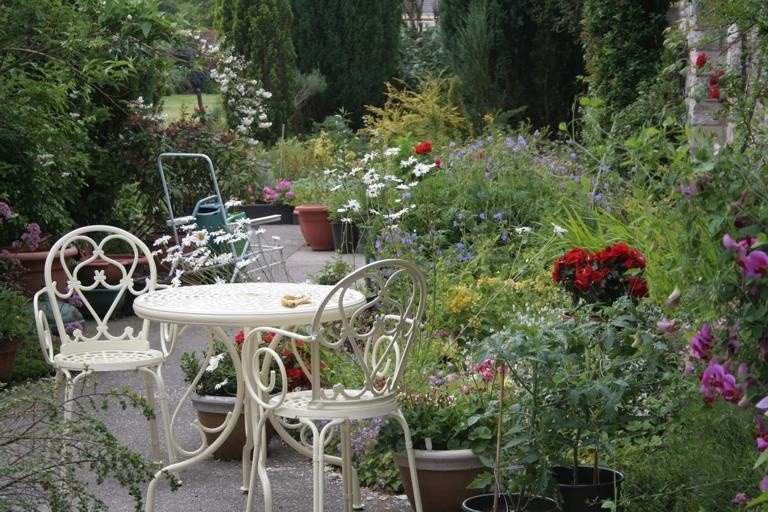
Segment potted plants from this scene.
[294,179,358,254]
[371,298,680,511]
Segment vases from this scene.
[0,236,195,387]
[192,393,274,460]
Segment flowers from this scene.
[181,330,324,395]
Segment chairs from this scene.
[33,225,183,496]
[157,153,286,284]
[242,258,426,512]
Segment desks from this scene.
[133,282,366,511]
